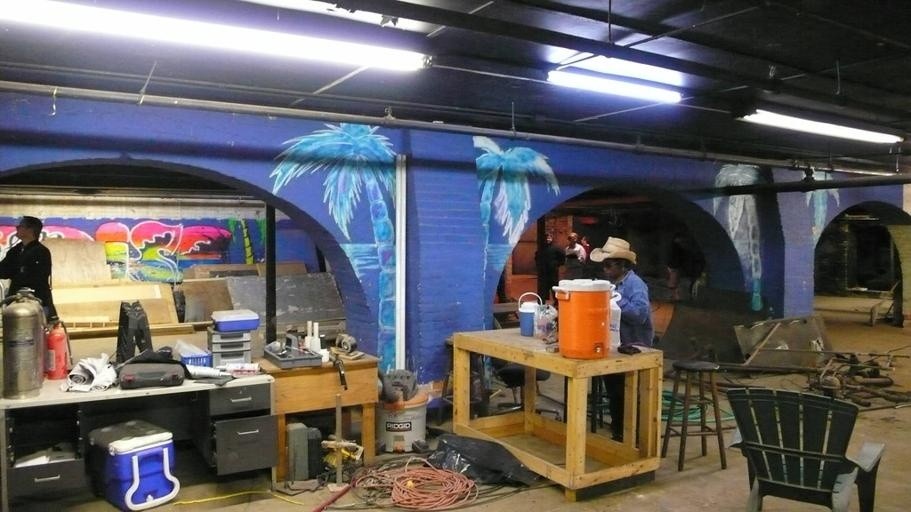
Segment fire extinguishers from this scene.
[44,313,73,382]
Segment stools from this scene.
[436,338,487,425]
[661,358,727,471]
[564,377,603,433]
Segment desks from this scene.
[252,348,382,483]
[0,374,279,512]
[453,328,664,503]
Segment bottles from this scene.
[310,322,322,350]
[304,319,313,350]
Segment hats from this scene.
[589,236,637,264]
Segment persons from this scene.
[580,234,594,261]
[0,217,56,319]
[665,232,685,302]
[535,231,565,306]
[591,235,654,443]
[563,232,585,279]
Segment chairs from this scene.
[725,388,885,512]
[492,318,562,422]
